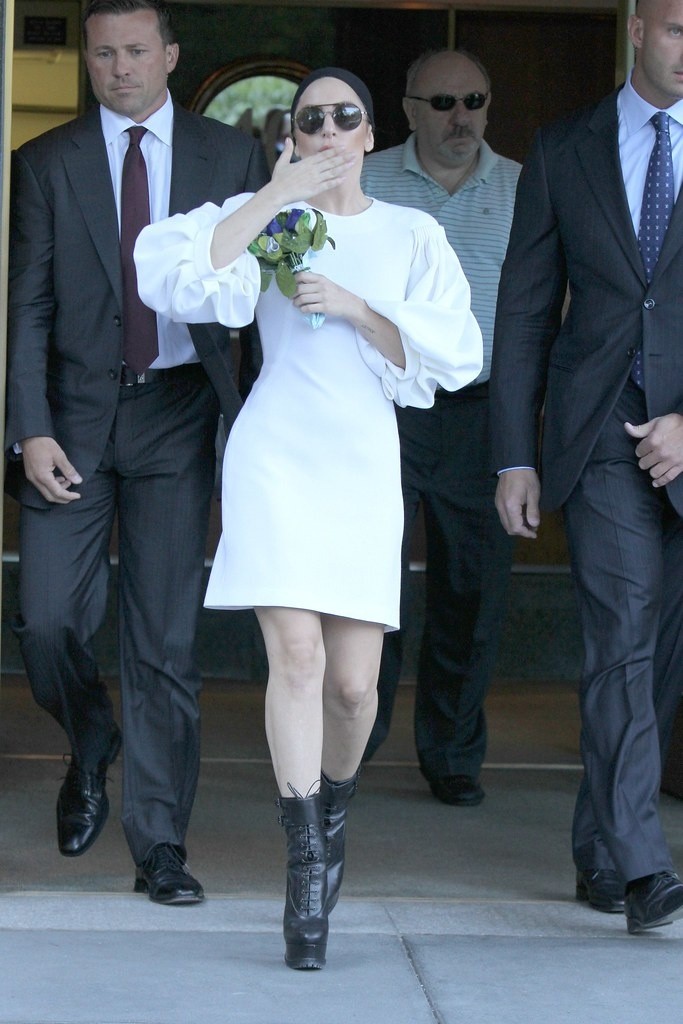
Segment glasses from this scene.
[408,92,489,112]
[293,101,367,135]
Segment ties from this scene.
[631,112,675,391]
[120,126,159,377]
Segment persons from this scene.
[4,0,272,904]
[488,0,683,933]
[134,50,570,970]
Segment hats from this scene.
[290,67,374,123]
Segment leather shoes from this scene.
[429,775,484,808]
[53,723,122,857]
[624,870,683,934]
[576,868,625,912]
[133,843,205,903]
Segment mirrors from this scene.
[193,60,315,142]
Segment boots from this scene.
[274,771,360,969]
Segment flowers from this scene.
[245,206,336,331]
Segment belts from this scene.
[435,389,488,403]
[121,366,177,387]
